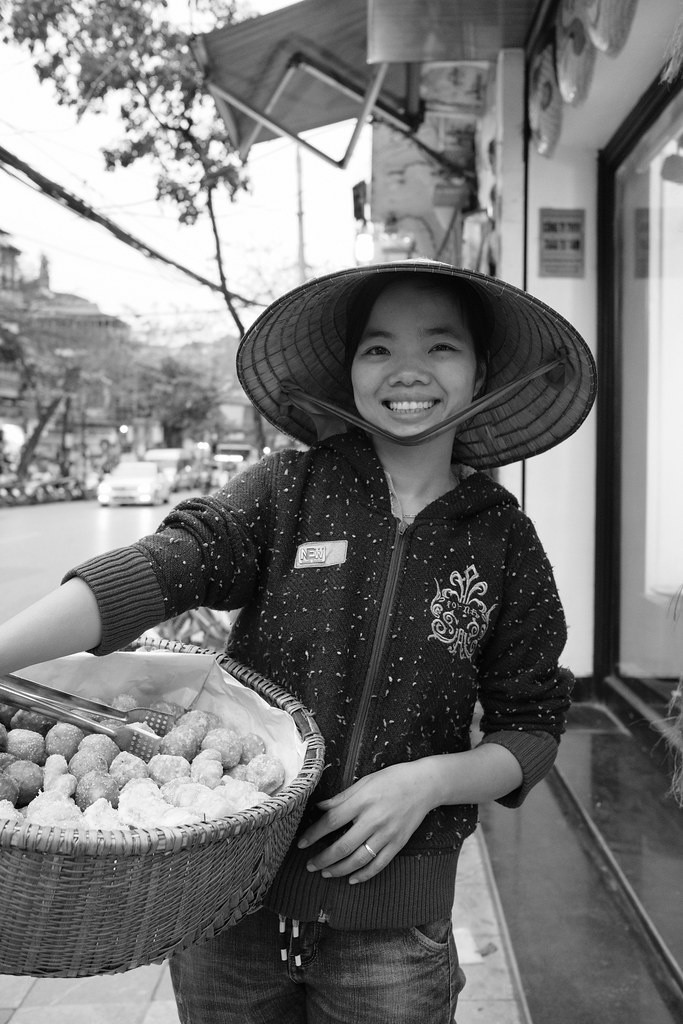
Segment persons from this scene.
[0,257,599,1024]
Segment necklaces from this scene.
[403,514,417,518]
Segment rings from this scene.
[364,842,376,858]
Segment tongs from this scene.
[0,672,174,763]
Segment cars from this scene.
[146,446,255,494]
[96,461,170,510]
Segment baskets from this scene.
[0,637,325,981]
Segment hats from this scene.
[235,256,598,470]
